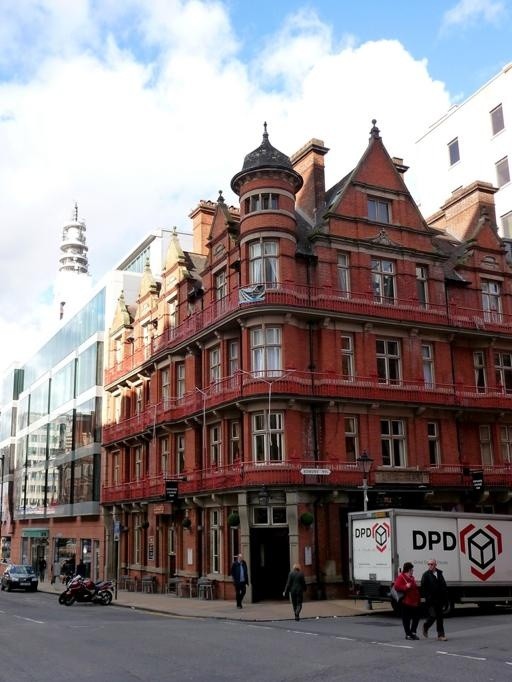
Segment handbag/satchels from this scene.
[390,586,404,602]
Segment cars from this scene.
[0,564,38,592]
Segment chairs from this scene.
[123,573,157,593]
[166,574,217,600]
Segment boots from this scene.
[295,606,301,619]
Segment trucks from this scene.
[348,508,512,616]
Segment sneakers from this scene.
[422,624,428,638]
[438,636,447,641]
[406,633,419,640]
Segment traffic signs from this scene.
[114,521,120,542]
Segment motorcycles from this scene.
[58,575,114,606]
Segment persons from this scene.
[230,553,249,609]
[282,562,308,621]
[420,559,449,641]
[61,559,74,585]
[50,560,57,585]
[394,561,419,640]
[76,558,86,578]
[38,557,47,583]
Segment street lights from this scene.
[357,449,374,611]
